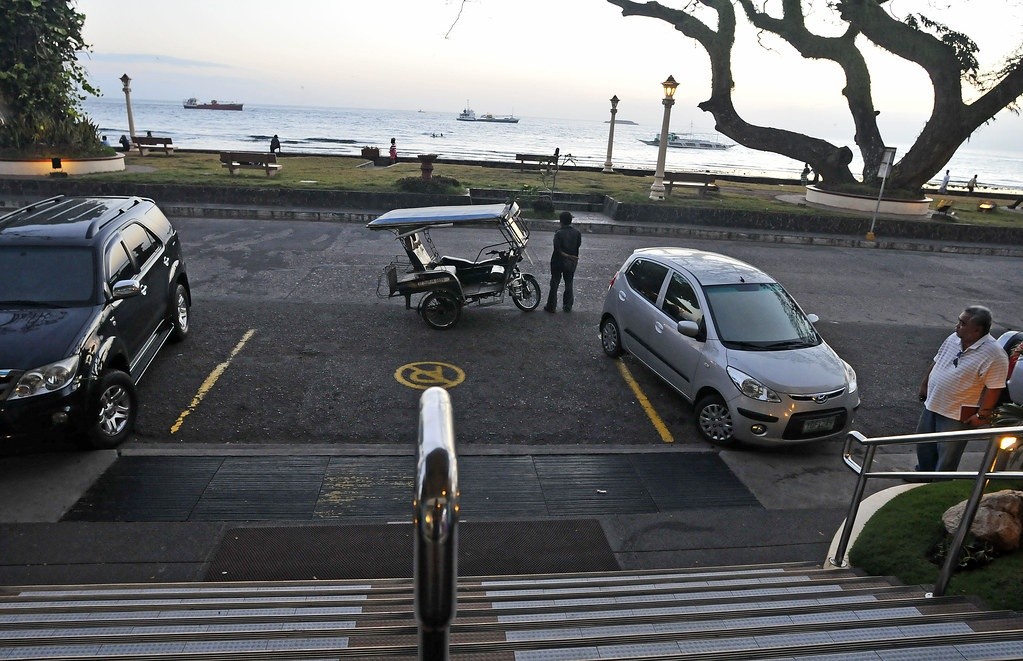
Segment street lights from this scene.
[649,75,680,202]
[119,73,138,147]
[600,94,621,174]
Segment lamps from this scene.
[51,158,62,172]
[937,205,951,216]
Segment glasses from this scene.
[953,351,962,368]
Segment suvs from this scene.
[0,194,193,451]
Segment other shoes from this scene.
[903,477,931,483]
[544,306,555,314]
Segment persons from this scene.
[968,175,979,193]
[903,306,1009,483]
[270,135,280,152]
[938,170,950,194]
[801,163,810,185]
[810,165,819,184]
[119,135,130,152]
[101,136,109,146]
[146,131,156,145]
[389,138,397,165]
[543,212,581,313]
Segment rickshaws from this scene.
[365,199,542,331]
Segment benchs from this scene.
[412,244,456,281]
[515,154,558,172]
[663,172,718,196]
[131,136,174,157]
[220,151,282,176]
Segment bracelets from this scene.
[976,413,986,420]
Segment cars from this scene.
[598,246,862,448]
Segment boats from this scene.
[182,96,244,111]
[456,99,521,124]
[636,131,736,151]
